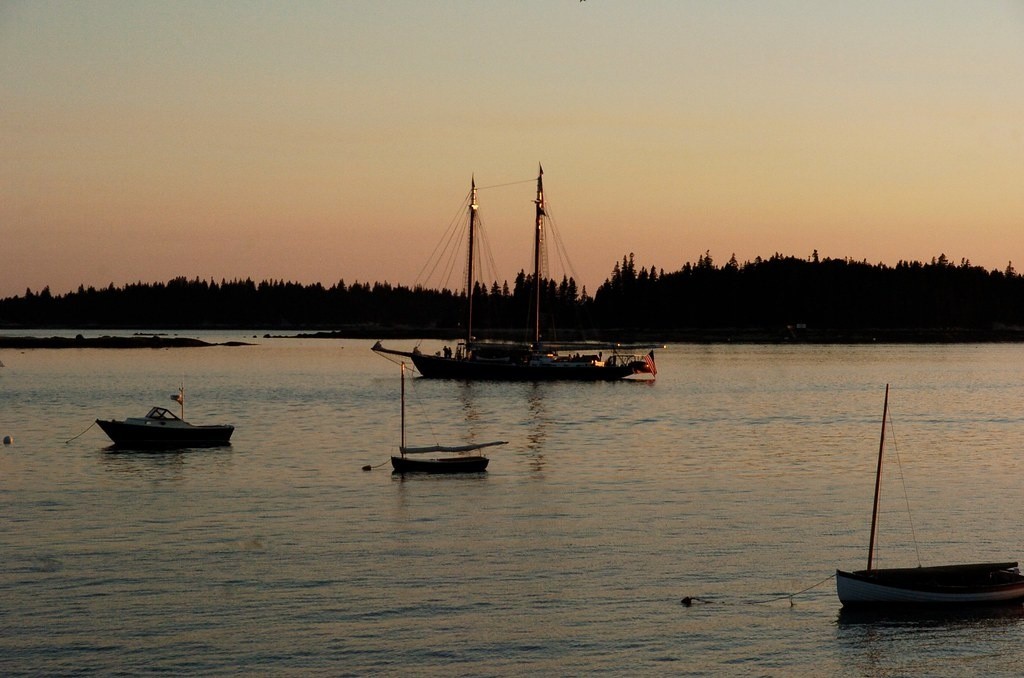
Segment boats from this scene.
[93,385,236,449]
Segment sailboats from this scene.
[835,381,1024,607]
[373,170,660,384]
[391,360,491,475]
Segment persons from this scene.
[443,345,459,359]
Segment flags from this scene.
[643,350,658,377]
